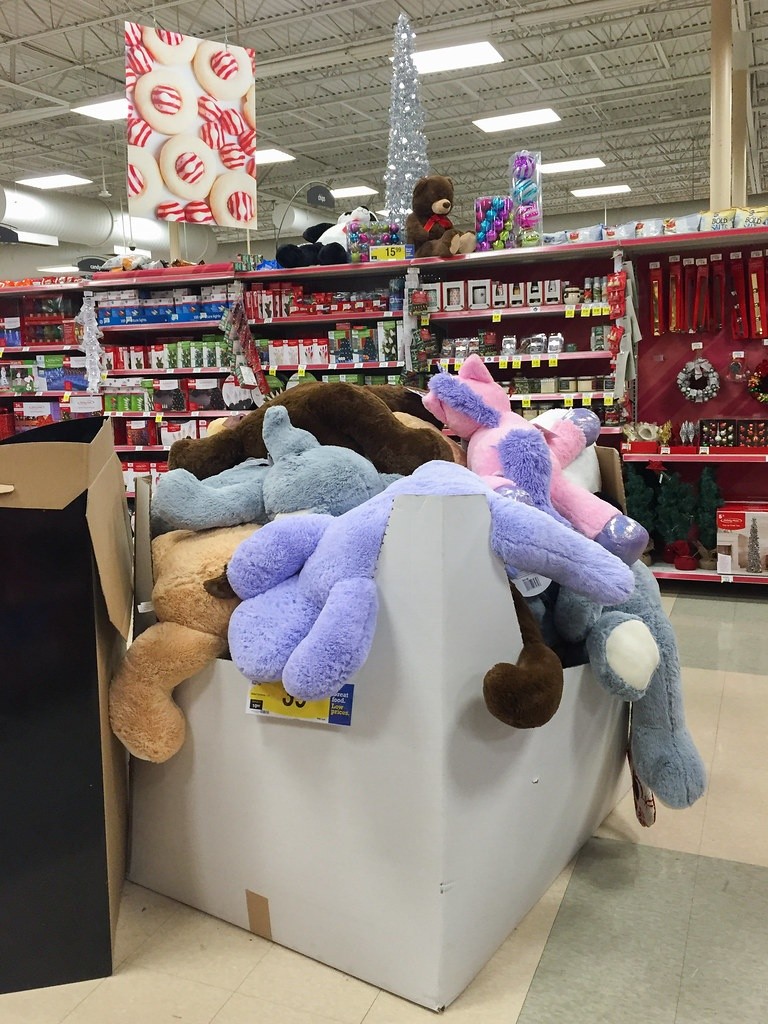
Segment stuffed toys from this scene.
[406,176,477,257]
[276,206,378,268]
[109,354,706,808]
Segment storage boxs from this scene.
[620,417,768,454]
[0,279,636,1013]
[715,499,768,578]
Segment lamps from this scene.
[10,91,379,199]
[388,37,633,197]
